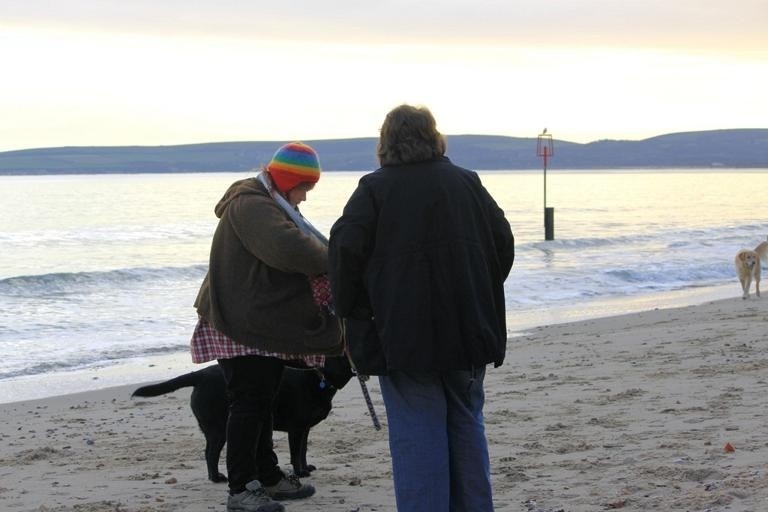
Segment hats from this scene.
[266,141,321,197]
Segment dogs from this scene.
[735,240,768,300]
[129,355,356,483]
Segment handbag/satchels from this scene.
[309,272,334,307]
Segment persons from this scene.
[189,141,351,512]
[327,105,515,509]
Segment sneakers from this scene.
[226,477,287,512]
[259,473,316,500]
